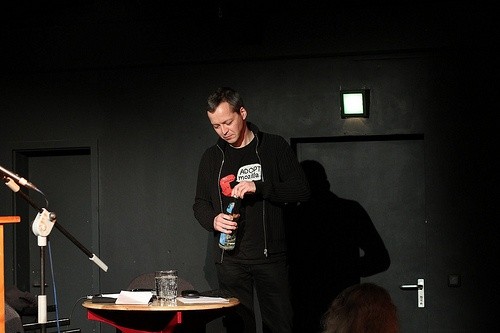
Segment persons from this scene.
[319,283,400,333]
[193,89,310,333]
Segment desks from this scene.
[82,294,240,333]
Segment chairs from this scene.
[128,273,196,295]
[5,283,81,333]
[324,283,400,333]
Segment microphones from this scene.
[0,166,36,191]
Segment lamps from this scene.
[340,89,369,119]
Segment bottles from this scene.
[217,195,239,250]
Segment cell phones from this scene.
[181,290,199,298]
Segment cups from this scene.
[155,270,178,302]
